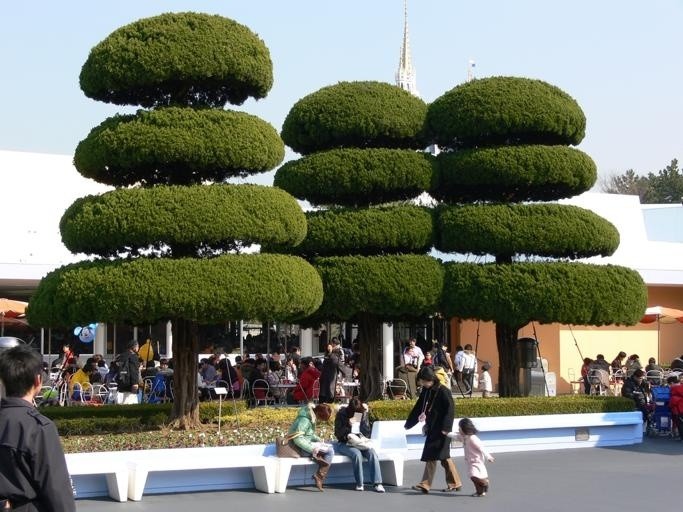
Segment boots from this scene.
[310,451,330,492]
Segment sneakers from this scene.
[374,484,385,492]
[355,484,364,490]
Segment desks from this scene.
[570,381,622,396]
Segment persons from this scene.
[0,346,79,512]
[441,418,495,497]
[288,402,333,493]
[580,351,682,442]
[404,367,461,494]
[1,333,494,407]
[333,397,386,493]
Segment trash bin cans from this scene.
[518,337,538,369]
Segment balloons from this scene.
[74,323,96,344]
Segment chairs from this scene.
[586,368,682,396]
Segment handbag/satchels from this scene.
[115,360,138,392]
[276,431,305,458]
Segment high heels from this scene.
[413,485,463,494]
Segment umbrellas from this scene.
[640,305,682,367]
[0,297,28,335]
